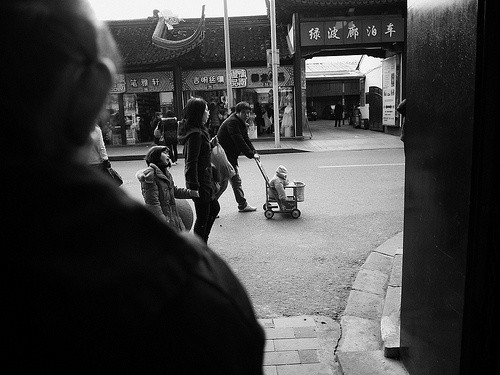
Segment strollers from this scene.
[254,155,306,220]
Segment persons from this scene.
[269,166,295,209]
[335,100,361,127]
[86,105,274,173]
[176,98,221,244]
[214,101,260,218]
[0,0,265,375]
[136,145,200,234]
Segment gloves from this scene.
[103,159,111,168]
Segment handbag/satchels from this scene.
[154,121,162,138]
[104,168,123,188]
[159,136,166,145]
[209,138,236,181]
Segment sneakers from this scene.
[238,204,257,212]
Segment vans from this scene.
[260,102,268,113]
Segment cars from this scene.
[307,105,318,121]
[326,104,336,120]
[348,105,360,125]
